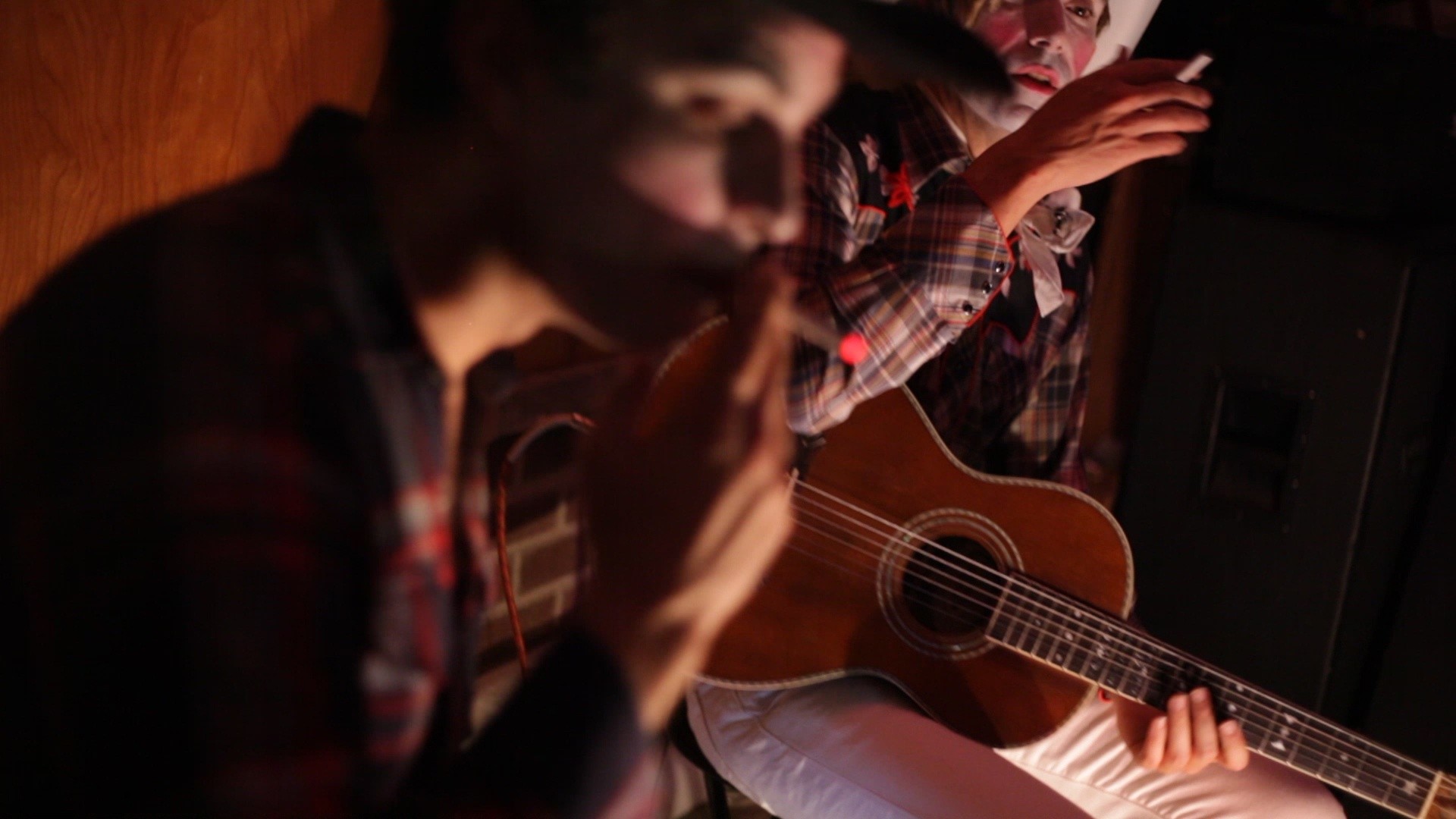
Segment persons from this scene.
[689,0,1345,819]
[0,0,1013,819]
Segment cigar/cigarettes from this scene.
[1175,54,1211,82]
[783,310,868,367]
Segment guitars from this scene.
[633,307,1456,819]
